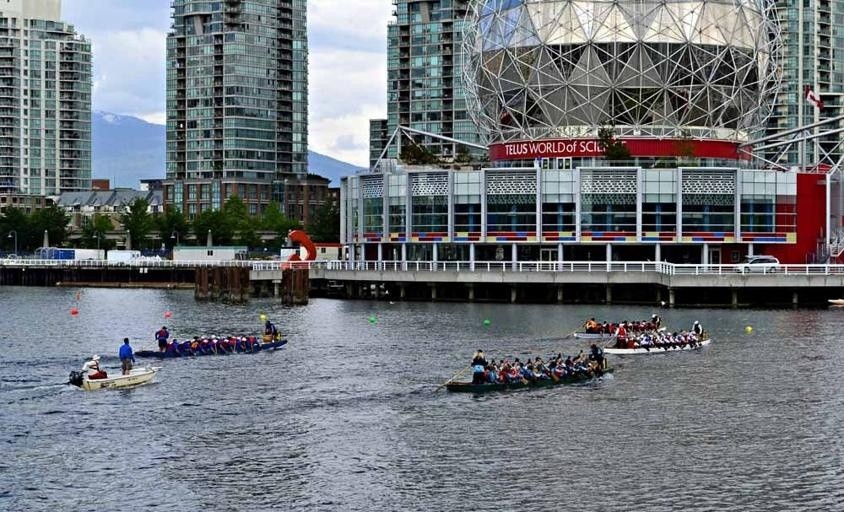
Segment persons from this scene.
[154,321,278,355]
[81,355,107,378]
[585,314,703,348]
[119,338,134,374]
[470,344,604,385]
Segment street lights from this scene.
[7,230,17,265]
[92,230,100,265]
[170,230,179,267]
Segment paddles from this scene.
[433,365,471,392]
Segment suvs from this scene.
[733,255,780,275]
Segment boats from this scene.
[133,337,292,359]
[572,326,666,340]
[601,336,711,356]
[445,367,614,398]
[67,366,161,392]
[827,298,844,305]
